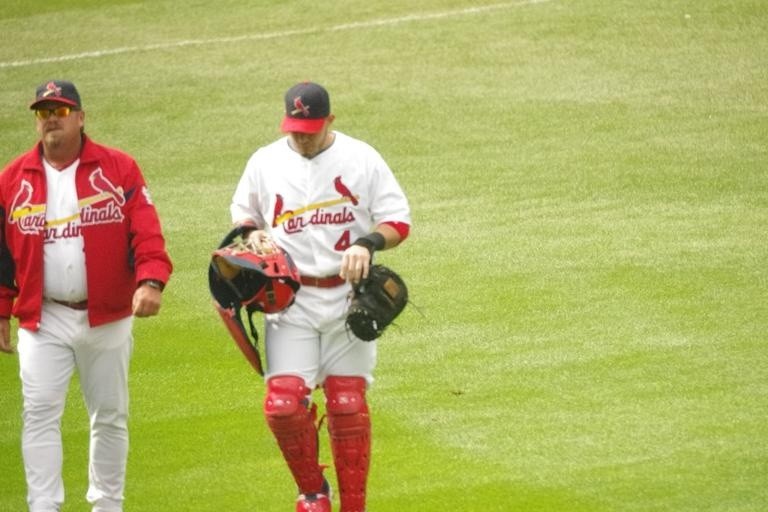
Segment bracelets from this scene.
[143,279,164,289]
[352,237,377,264]
[364,231,386,251]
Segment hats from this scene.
[30,79,82,109]
[280,80,331,135]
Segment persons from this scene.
[231,80,414,511]
[1,76,175,511]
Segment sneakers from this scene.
[295,479,332,512]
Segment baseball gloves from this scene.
[346,265,409,342]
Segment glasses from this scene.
[36,105,70,120]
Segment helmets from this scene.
[208,237,302,313]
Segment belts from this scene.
[301,275,346,288]
[53,299,87,311]
